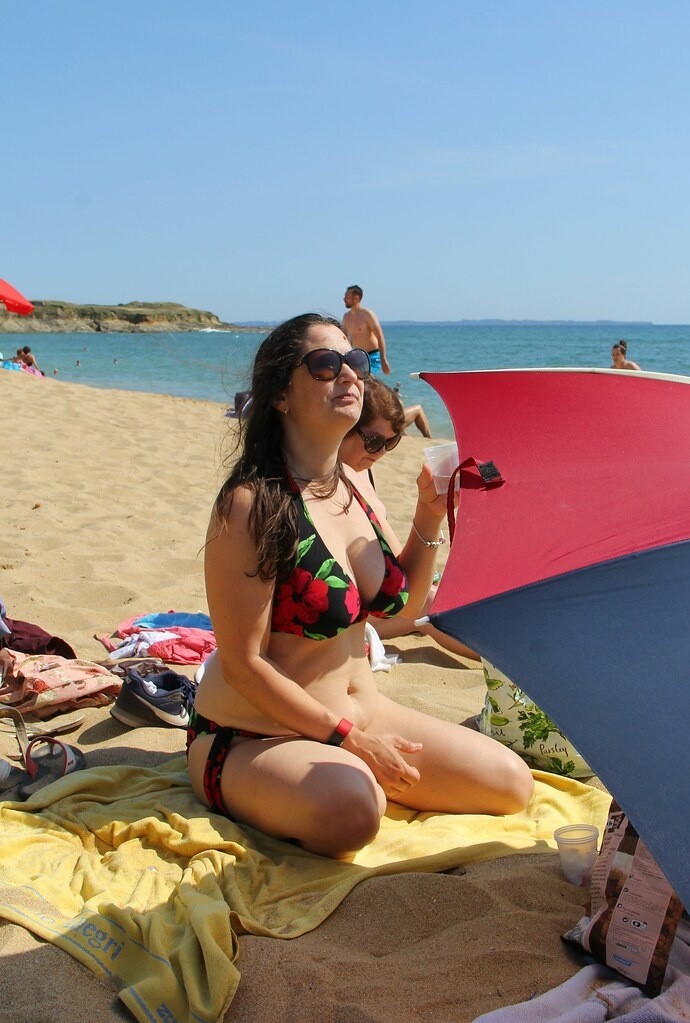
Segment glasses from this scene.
[294,349,370,381]
[354,424,402,454]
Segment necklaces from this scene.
[288,460,350,515]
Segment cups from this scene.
[421,442,460,495]
[554,824,600,886]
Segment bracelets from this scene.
[412,518,446,548]
[327,718,353,746]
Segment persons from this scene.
[338,370,487,662]
[398,401,432,438]
[610,340,640,371]
[13,345,45,376]
[342,285,391,379]
[391,387,399,395]
[76,360,80,366]
[114,359,117,363]
[185,309,535,858]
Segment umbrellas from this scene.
[409,362,690,921]
[0,279,36,316]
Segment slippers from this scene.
[0,707,86,739]
[16,736,86,801]
[0,757,25,790]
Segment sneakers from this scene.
[111,670,199,729]
